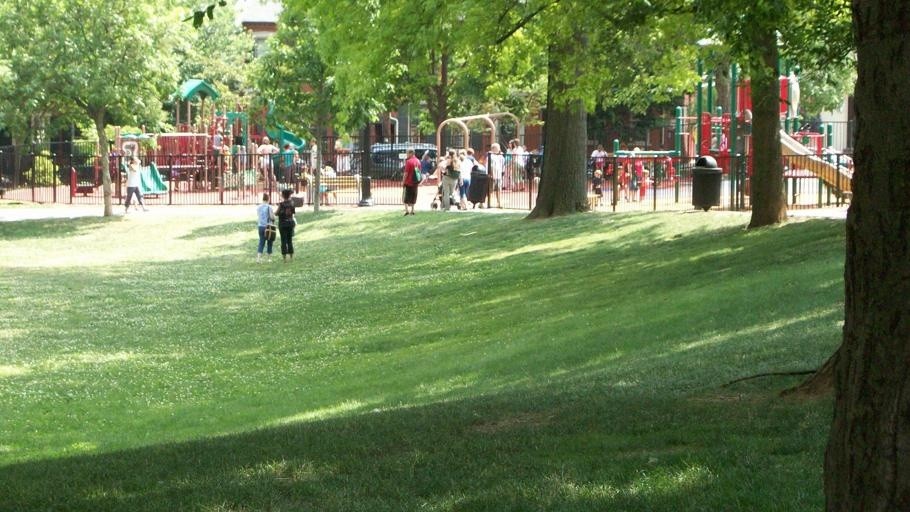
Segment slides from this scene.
[779,129,852,192]
[256,102,305,180]
[124,162,167,196]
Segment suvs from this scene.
[351,140,437,180]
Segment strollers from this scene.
[431,171,461,212]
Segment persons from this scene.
[124,156,149,214]
[486,138,545,192]
[255,188,297,259]
[320,160,338,206]
[591,144,644,202]
[212,133,317,195]
[402,147,479,215]
[170,155,180,191]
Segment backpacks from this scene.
[409,158,422,183]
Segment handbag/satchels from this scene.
[448,169,460,178]
[264,224,276,241]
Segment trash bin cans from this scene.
[468,164,489,209]
[691,155,723,212]
